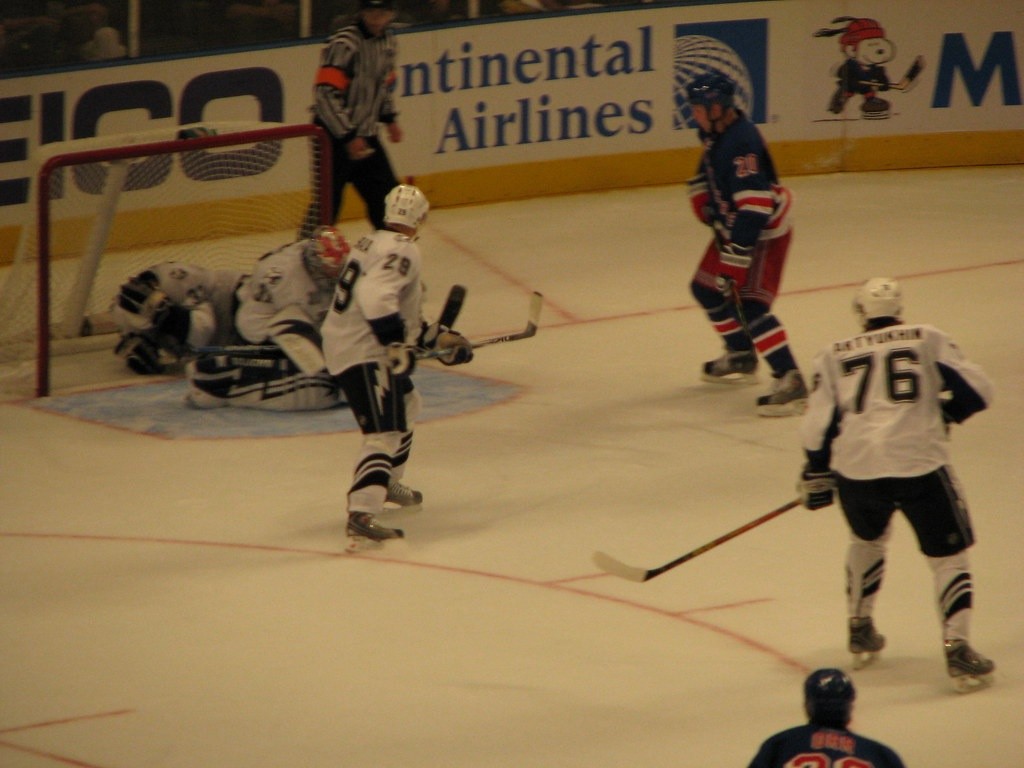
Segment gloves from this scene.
[714,252,751,297]
[385,343,417,379]
[686,174,718,227]
[422,324,476,367]
[796,469,836,510]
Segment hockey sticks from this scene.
[187,285,466,358]
[707,206,749,337]
[590,497,804,583]
[407,291,545,356]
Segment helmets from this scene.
[384,185,431,232]
[853,277,904,323]
[803,668,856,704]
[685,72,738,108]
[308,226,351,276]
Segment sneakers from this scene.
[383,481,424,514]
[944,638,997,694]
[342,512,406,554]
[703,355,760,383]
[755,371,810,416]
[846,616,888,670]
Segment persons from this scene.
[296,0,404,241]
[186,226,351,411]
[321,184,475,540]
[745,665,905,768]
[82,25,129,67]
[109,262,233,376]
[686,71,815,418]
[794,277,995,695]
